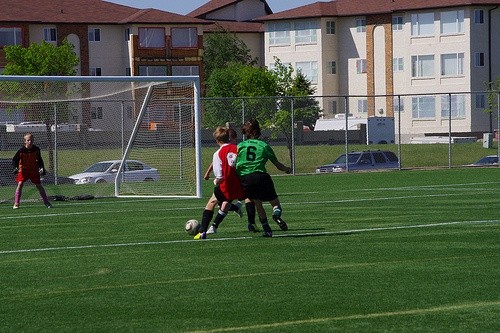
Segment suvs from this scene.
[315,149,402,173]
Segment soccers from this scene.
[184,220,200,234]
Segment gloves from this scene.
[13,170,18,175]
[39,168,47,175]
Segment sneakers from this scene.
[249,224,262,232]
[13,203,19,210]
[271,213,288,231]
[206,226,217,234]
[236,201,244,218]
[193,232,207,239]
[263,232,273,237]
[47,204,54,208]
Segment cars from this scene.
[0,158,76,187]
[469,156,500,167]
[69,160,159,186]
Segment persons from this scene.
[193,127,261,240]
[11,133,54,209]
[235,119,293,237]
